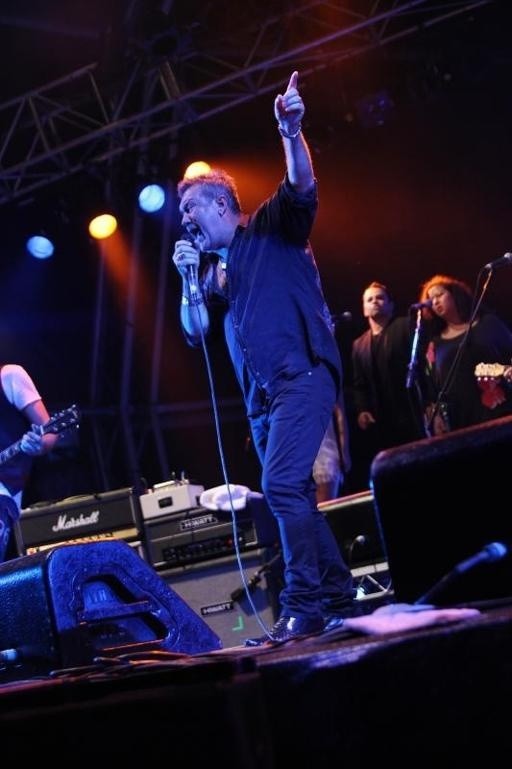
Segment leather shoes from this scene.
[243,614,323,645]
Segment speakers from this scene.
[2,537,223,670]
[367,413,511,611]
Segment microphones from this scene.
[454,539,507,581]
[230,571,262,603]
[409,298,434,314]
[483,250,511,272]
[328,311,352,324]
[178,232,208,303]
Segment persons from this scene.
[172,70,365,648]
[415,275,512,435]
[310,401,353,505]
[351,280,431,459]
[0,362,58,565]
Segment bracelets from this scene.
[180,294,204,307]
[277,123,303,139]
